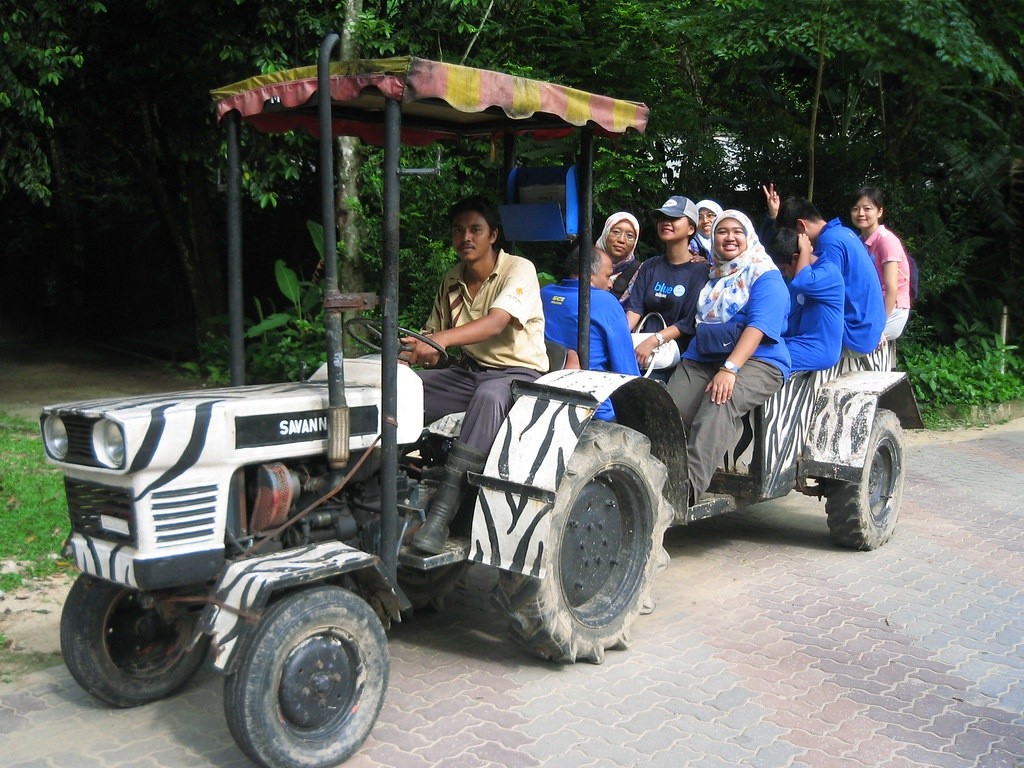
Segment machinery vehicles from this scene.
[36,33,926,768]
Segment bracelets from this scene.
[719,367,738,379]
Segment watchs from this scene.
[654,332,664,345]
[723,360,740,373]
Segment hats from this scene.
[651,195,699,228]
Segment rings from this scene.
[424,361,429,364]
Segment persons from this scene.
[536,183,912,507]
[405,197,549,555]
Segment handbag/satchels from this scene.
[630,312,681,370]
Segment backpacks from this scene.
[898,256,918,298]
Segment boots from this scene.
[412,438,490,555]
